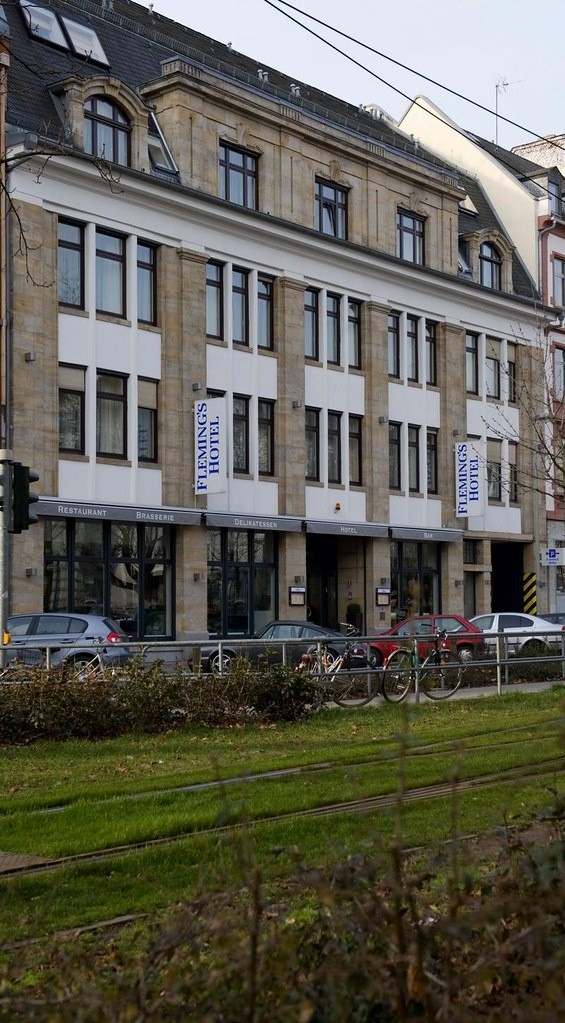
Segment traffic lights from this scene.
[12,461,39,534]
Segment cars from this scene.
[449,612,565,657]
[354,614,484,665]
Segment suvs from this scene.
[6,613,131,680]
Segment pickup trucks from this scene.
[188,618,371,671]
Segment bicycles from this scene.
[0,635,130,683]
[290,623,381,711]
[381,620,466,703]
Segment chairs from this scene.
[288,627,298,638]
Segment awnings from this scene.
[29,496,202,526]
[203,508,303,532]
[304,517,390,538]
[390,524,464,543]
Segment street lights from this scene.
[533,412,556,614]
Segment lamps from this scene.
[25,350,38,363]
[295,575,305,584]
[193,381,202,392]
[454,428,462,435]
[25,567,37,578]
[455,579,461,586]
[380,577,389,585]
[193,572,205,582]
[292,400,302,408]
[539,581,545,589]
[380,415,388,424]
